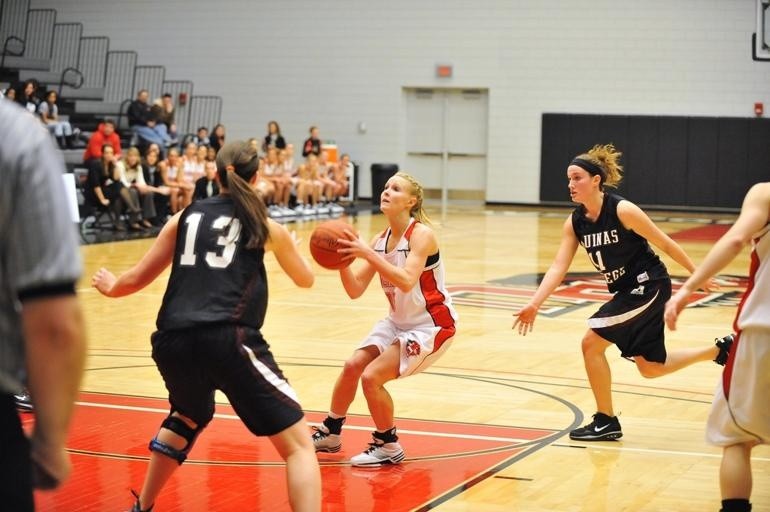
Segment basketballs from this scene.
[310,220,360,271]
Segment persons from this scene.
[513,145,737,441]
[0,95,85,512]
[193,164,215,201]
[138,143,170,227]
[2,83,75,147]
[89,147,138,233]
[92,139,321,512]
[181,144,198,199]
[250,120,353,216]
[83,92,228,156]
[194,142,206,193]
[117,147,155,230]
[160,147,186,216]
[664,182,766,512]
[311,172,460,467]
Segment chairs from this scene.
[76,172,116,231]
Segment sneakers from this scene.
[311,422,343,453]
[712,333,736,366]
[129,223,145,231]
[13,393,35,412]
[569,411,623,441]
[350,436,406,467]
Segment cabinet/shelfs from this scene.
[598,115,658,206]
[540,113,598,203]
[719,117,770,208]
[660,116,719,206]
